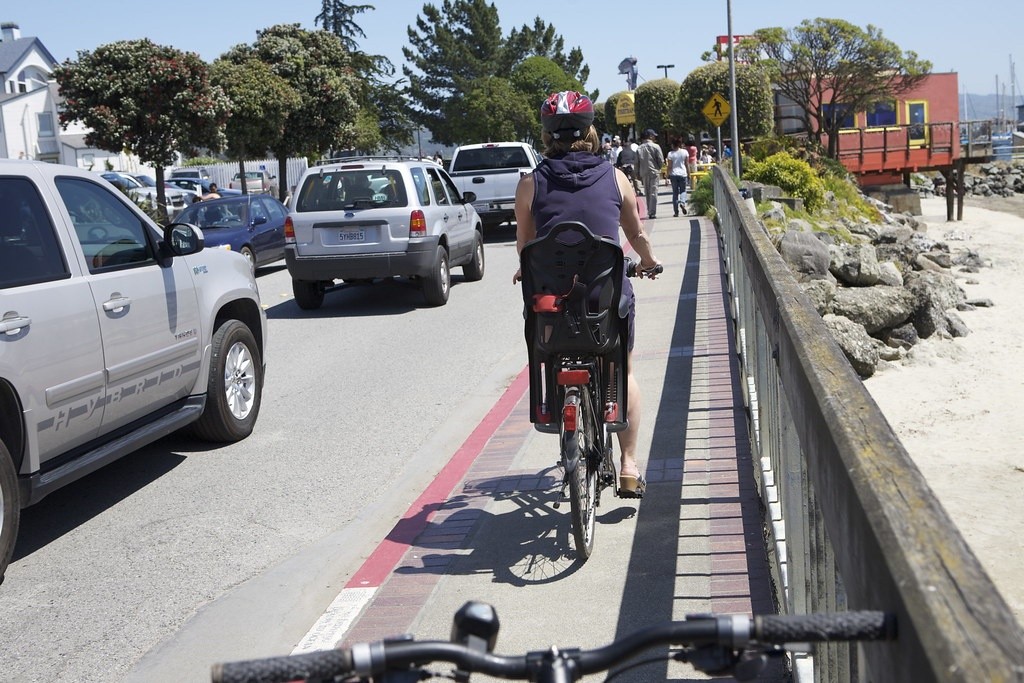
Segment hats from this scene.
[645,130,657,136]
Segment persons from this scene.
[685,139,698,183]
[665,139,690,217]
[601,135,643,195]
[433,151,444,166]
[512,90,661,493]
[192,183,225,223]
[699,143,745,171]
[633,129,665,219]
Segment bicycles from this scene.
[516,257,664,560]
[211,600,899,683]
[613,162,640,214]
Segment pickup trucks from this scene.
[447,142,545,231]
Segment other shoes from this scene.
[649,214,656,218]
[680,203,687,214]
[674,214,678,217]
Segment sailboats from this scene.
[956,54,1024,148]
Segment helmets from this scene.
[540,90,594,141]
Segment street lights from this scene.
[657,63,674,80]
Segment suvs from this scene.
[229,172,277,197]
[283,155,485,310]
[0,159,268,587]
[91,170,188,221]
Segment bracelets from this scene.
[639,259,657,272]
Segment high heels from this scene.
[620,468,646,497]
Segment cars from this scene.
[172,194,289,278]
[169,167,209,182]
[165,177,256,199]
